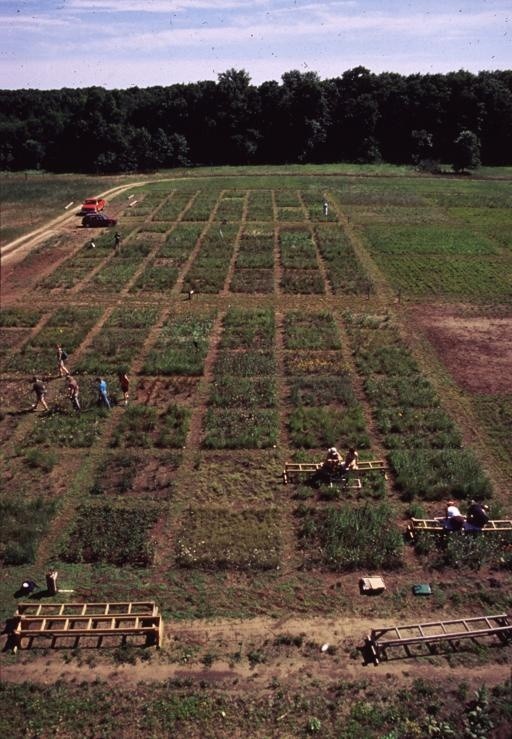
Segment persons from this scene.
[339,448,358,473]
[31,376,49,411]
[310,448,342,487]
[118,372,130,406]
[55,343,70,376]
[114,232,120,248]
[96,377,112,410]
[445,502,465,536]
[322,194,328,216]
[467,499,488,524]
[66,375,82,411]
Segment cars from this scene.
[81,198,106,213]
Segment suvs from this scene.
[81,212,118,228]
